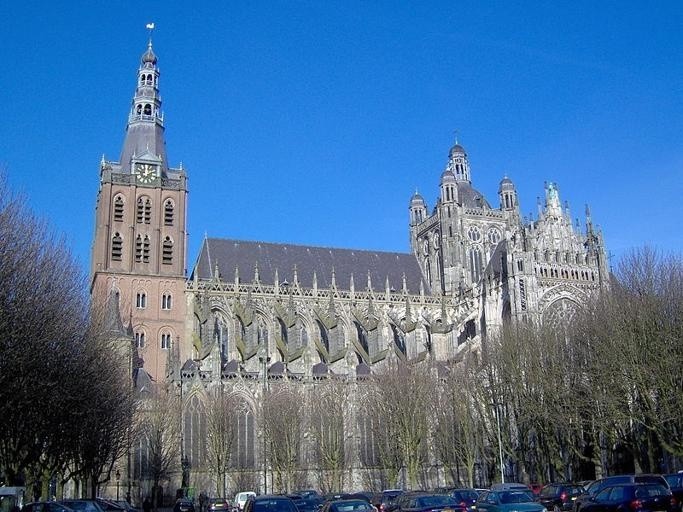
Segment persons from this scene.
[143,497,154,512]
[52,494,56,502]
[126,494,131,505]
[199,493,204,512]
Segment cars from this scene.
[24,498,140,512]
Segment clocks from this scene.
[136,163,157,184]
